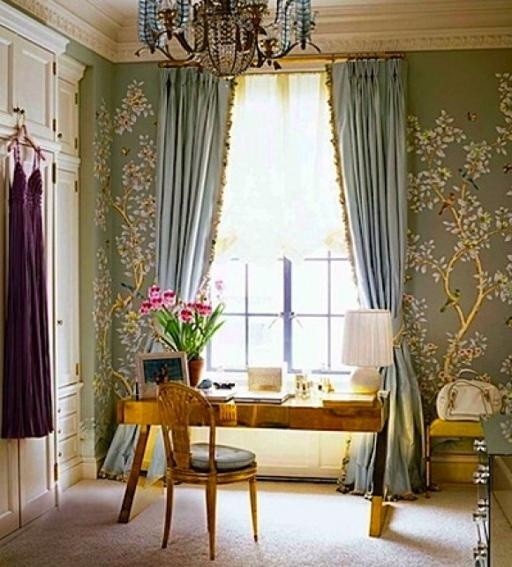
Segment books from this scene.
[323,392,376,408]
[207,390,290,404]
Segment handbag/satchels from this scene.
[436,379,502,421]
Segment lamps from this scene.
[340,307,395,394]
[133,1,322,81]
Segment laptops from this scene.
[234,392,290,403]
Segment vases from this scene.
[189,357,205,399]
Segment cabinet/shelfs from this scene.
[0,2,90,542]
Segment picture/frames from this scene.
[136,352,188,401]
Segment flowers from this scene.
[138,284,228,363]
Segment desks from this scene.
[113,385,393,538]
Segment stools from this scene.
[426,418,485,492]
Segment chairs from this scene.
[156,380,261,560]
